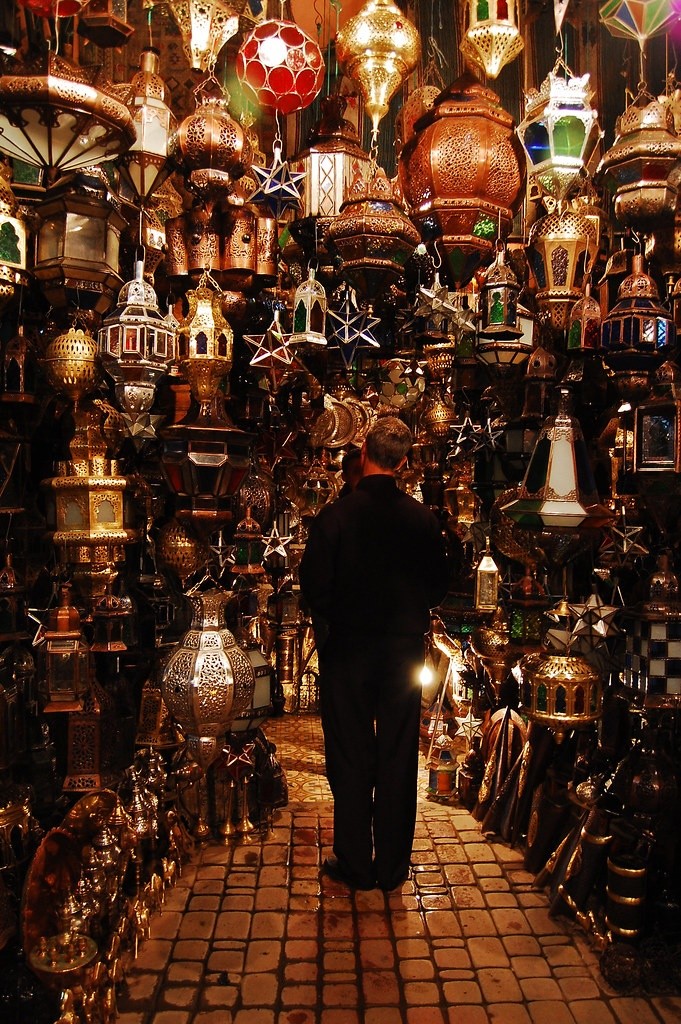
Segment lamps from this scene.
[0,0,681,1024]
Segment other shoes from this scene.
[374,857,409,891]
[325,855,376,891]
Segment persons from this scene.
[297,418,448,891]
